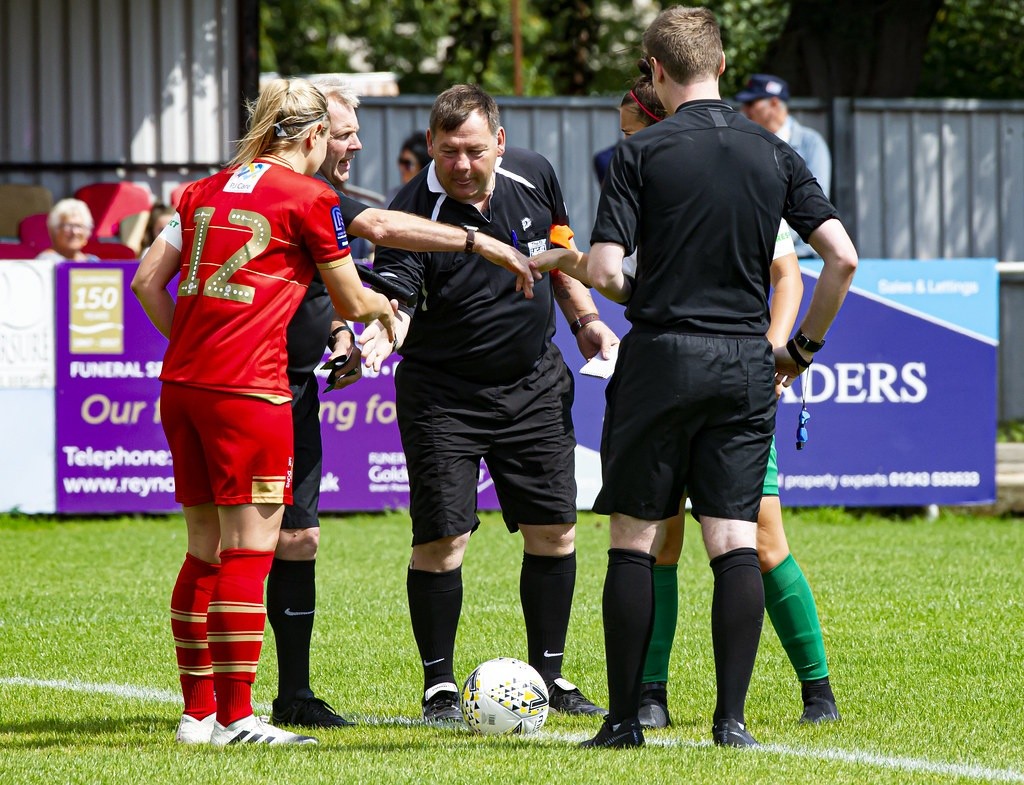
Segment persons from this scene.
[129,74,854,265]
[355,84,622,720]
[38,197,101,265]
[128,75,545,759]
[515,6,860,755]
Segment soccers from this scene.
[461,656,550,736]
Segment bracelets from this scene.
[328,327,356,352]
[570,313,600,334]
[787,338,814,367]
[794,327,826,353]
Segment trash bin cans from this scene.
[999,270,1024,422]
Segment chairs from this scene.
[0,179,198,261]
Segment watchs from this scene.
[463,223,479,254]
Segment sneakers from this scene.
[272,698,355,727]
[210,714,318,745]
[799,697,842,726]
[637,699,671,728]
[712,718,760,747]
[421,676,463,722]
[176,713,216,744]
[544,673,609,717]
[578,714,647,749]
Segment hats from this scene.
[735,74,789,102]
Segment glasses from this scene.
[742,97,768,107]
[398,157,420,168]
[56,224,87,233]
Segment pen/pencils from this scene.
[511,229,518,250]
